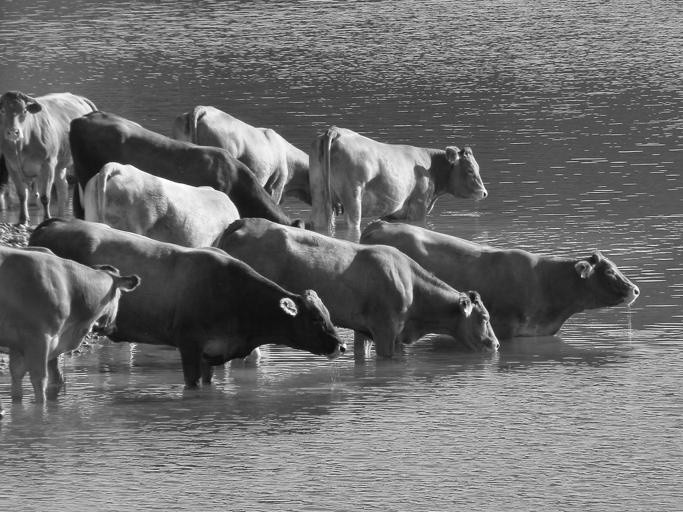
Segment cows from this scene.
[69,109,303,232]
[358,218,641,340]
[0,150,61,209]
[209,216,502,365]
[173,101,313,205]
[0,89,99,223]
[307,123,489,229]
[28,217,348,390]
[81,161,241,250]
[0,242,144,405]
